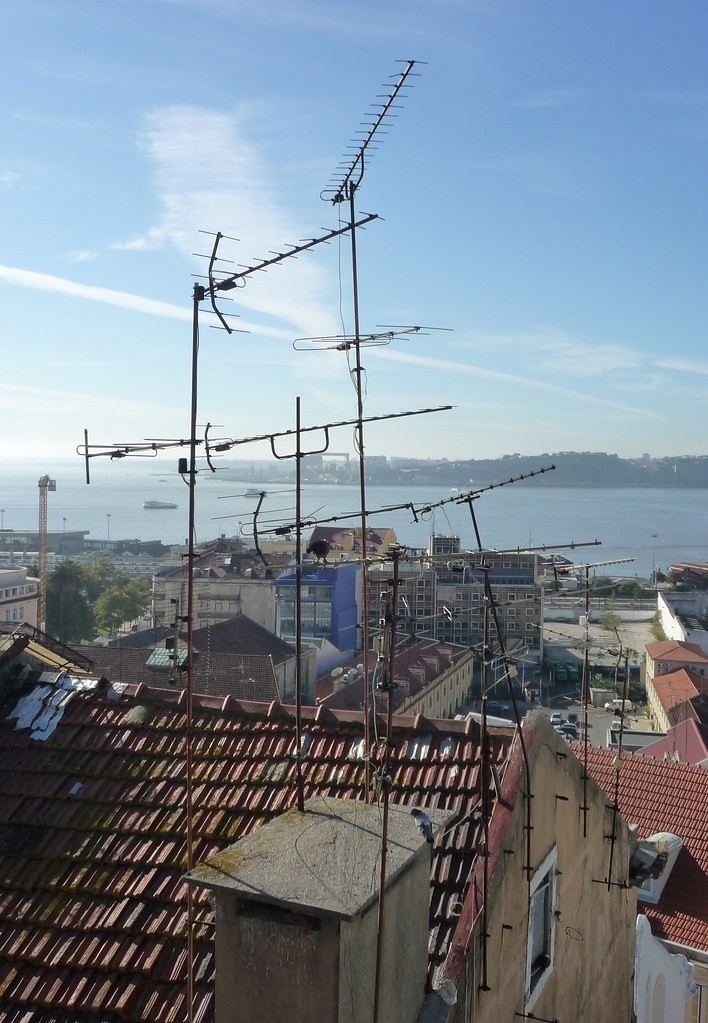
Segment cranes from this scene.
[37,475,56,634]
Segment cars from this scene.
[567,712,578,724]
[605,699,632,712]
[550,713,562,725]
[574,599,591,607]
[487,701,509,711]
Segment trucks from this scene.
[563,659,581,682]
[542,608,576,624]
[545,659,568,682]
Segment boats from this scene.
[143,501,177,509]
[449,488,461,492]
[245,488,260,497]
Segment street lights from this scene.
[651,534,658,590]
[1,509,5,529]
[62,517,67,555]
[107,513,111,540]
